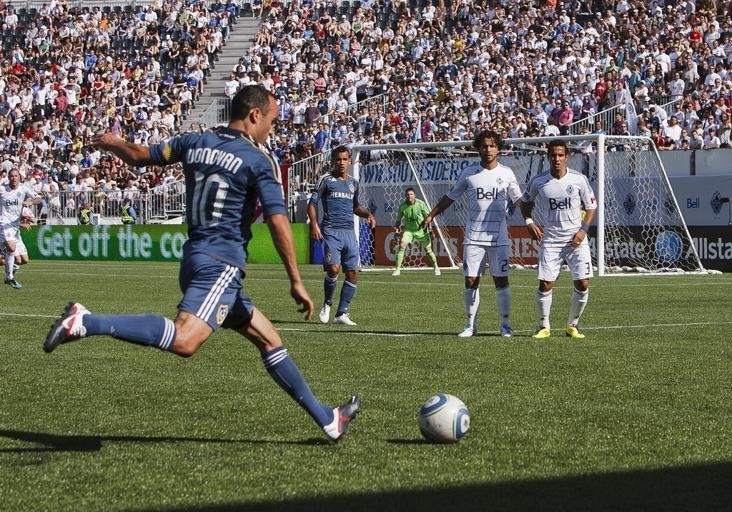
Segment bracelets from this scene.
[525,218,534,226]
[582,223,590,233]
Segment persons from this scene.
[520,139,599,339]
[43,84,362,443]
[306,145,376,326]
[0,168,41,290]
[392,187,442,278]
[2,1,731,225]
[418,130,535,339]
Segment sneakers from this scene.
[457,324,586,338]
[322,396,363,444]
[333,313,357,326]
[319,303,331,324]
[43,302,92,353]
[435,268,441,276]
[392,271,400,276]
[4,278,22,289]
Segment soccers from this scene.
[656,231,683,261]
[420,392,471,443]
